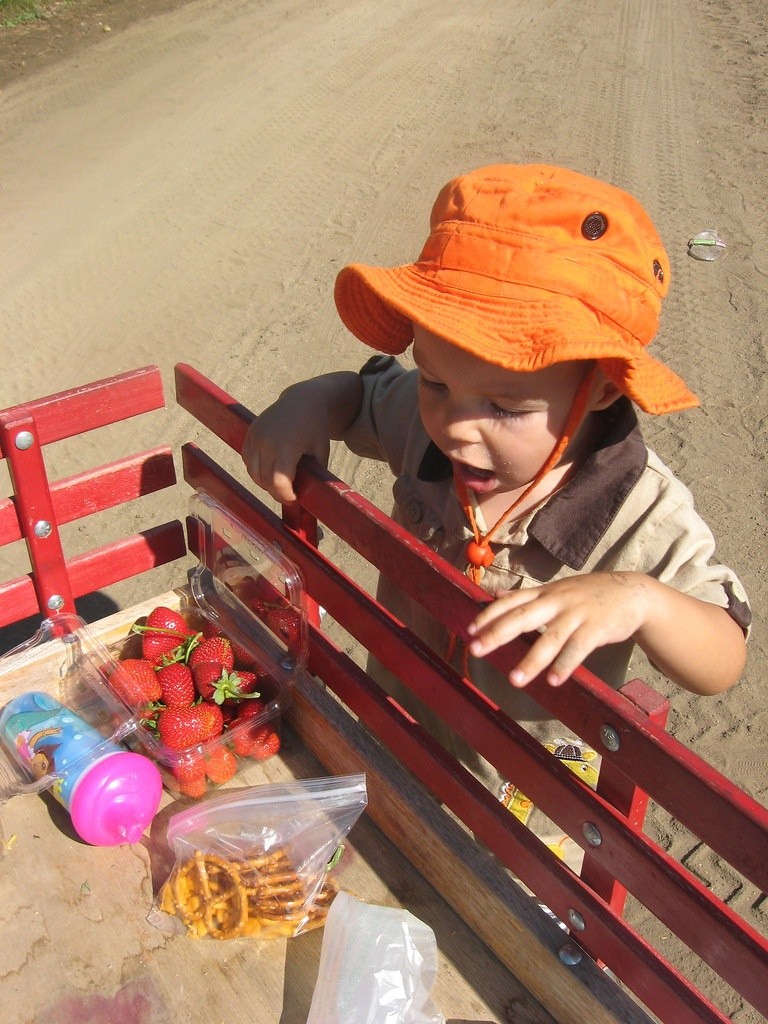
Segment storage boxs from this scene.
[0,614,155,796]
[78,489,307,805]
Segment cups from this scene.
[0,691,163,847]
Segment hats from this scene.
[334,163,697,411]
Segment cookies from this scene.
[161,840,345,940]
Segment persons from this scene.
[241,163,753,935]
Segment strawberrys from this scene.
[97,605,281,799]
